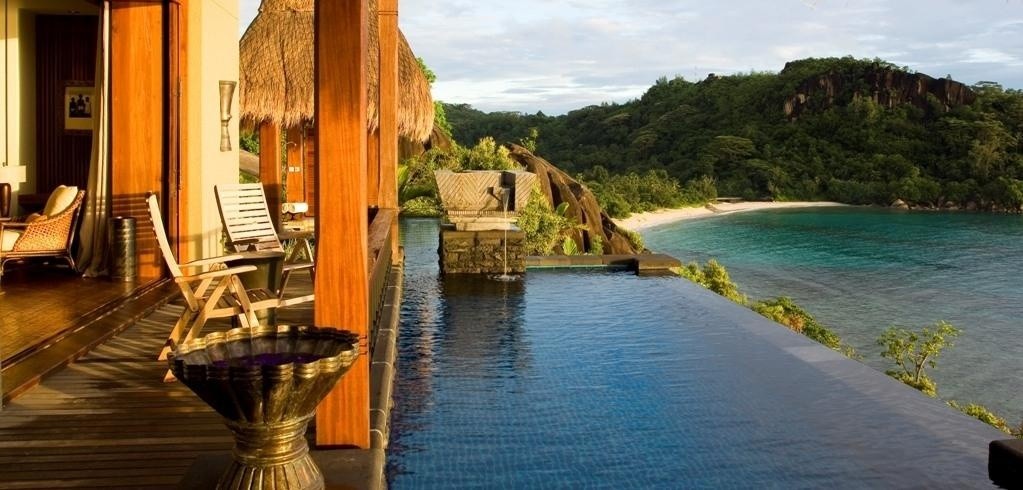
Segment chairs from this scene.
[0,184,87,277]
[146,181,316,382]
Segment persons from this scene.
[70,93,92,118]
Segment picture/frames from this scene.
[63,87,96,131]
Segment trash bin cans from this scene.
[108,216,135,281]
